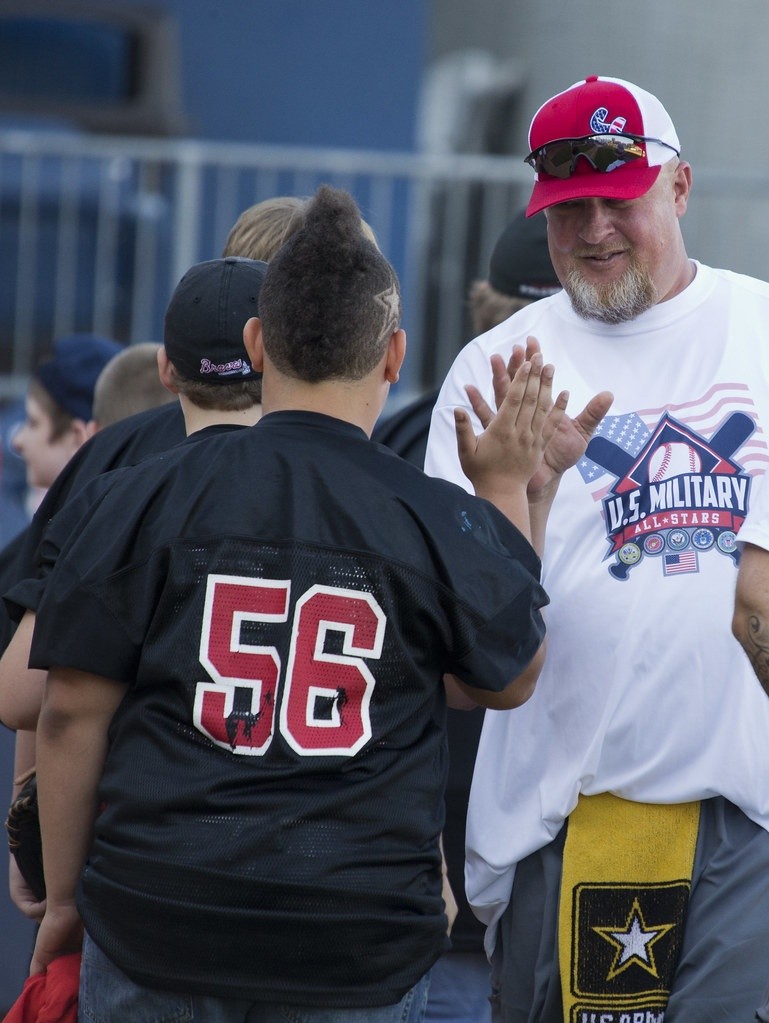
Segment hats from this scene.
[166,257,269,387]
[490,204,564,303]
[524,75,682,221]
[34,331,126,422]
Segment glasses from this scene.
[521,131,681,179]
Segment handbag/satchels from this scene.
[6,760,48,902]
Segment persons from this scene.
[0,186,570,1023]
[361,69,768,1023]
